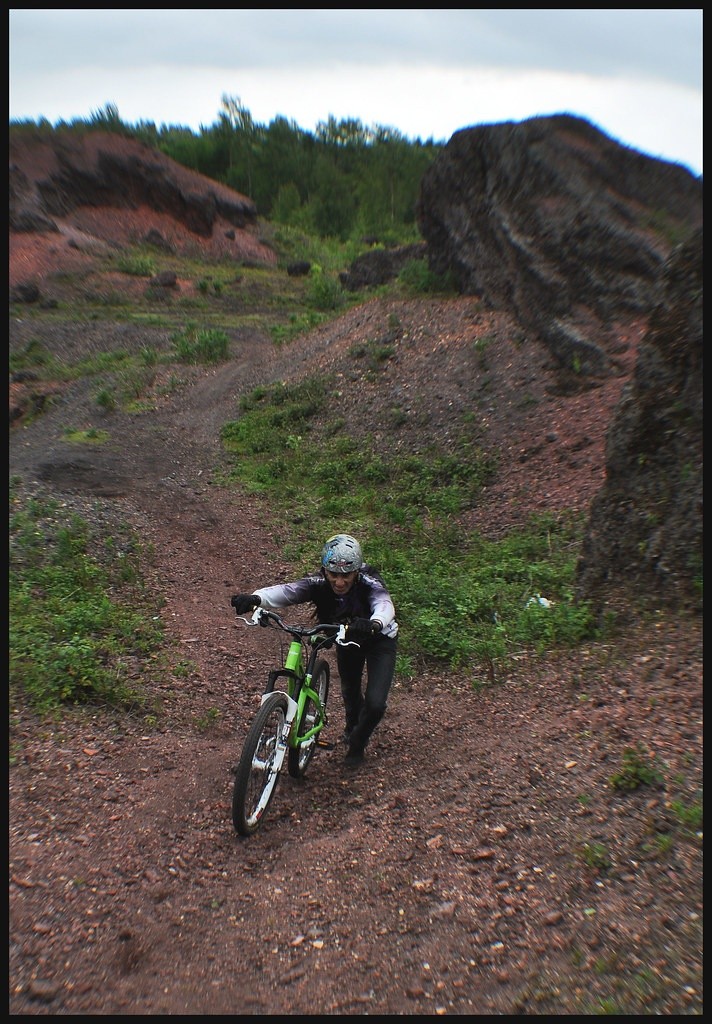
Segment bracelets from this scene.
[372,619,382,630]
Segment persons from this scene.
[231,534,399,770]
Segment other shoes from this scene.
[344,719,359,737]
[345,743,364,769]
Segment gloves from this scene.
[347,617,380,645]
[231,593,261,615]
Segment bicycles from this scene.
[231,605,376,838]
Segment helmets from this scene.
[321,534,362,573]
